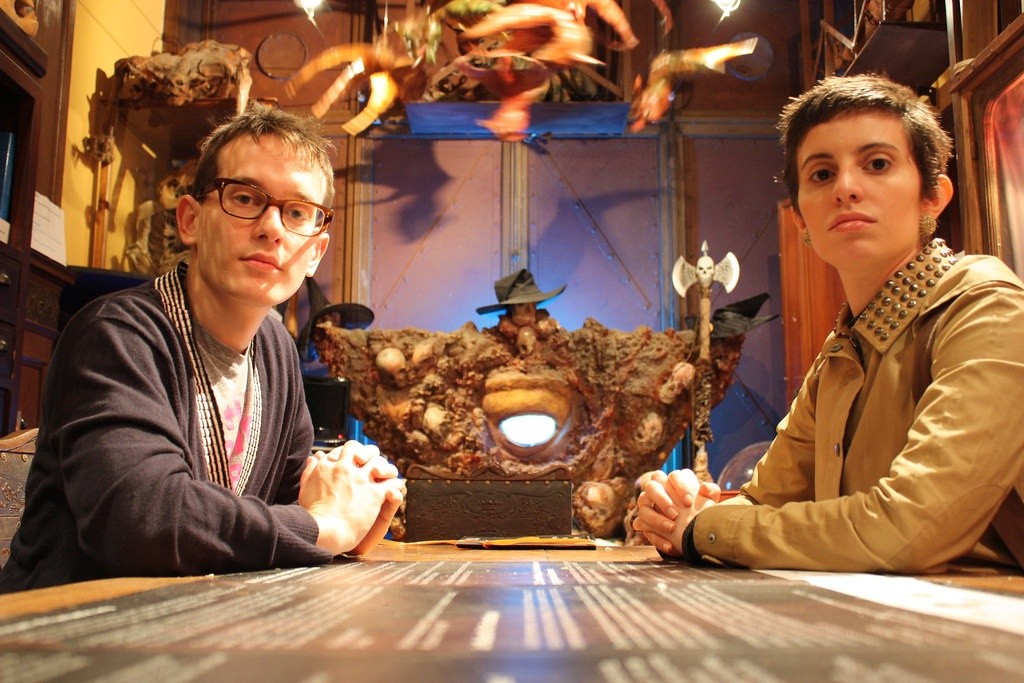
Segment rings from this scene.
[651,501,655,509]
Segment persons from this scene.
[0,102,406,594]
[632,75,1024,575]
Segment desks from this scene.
[0,545,1024,683]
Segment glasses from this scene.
[195,177,334,237]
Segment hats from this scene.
[476,268,567,315]
